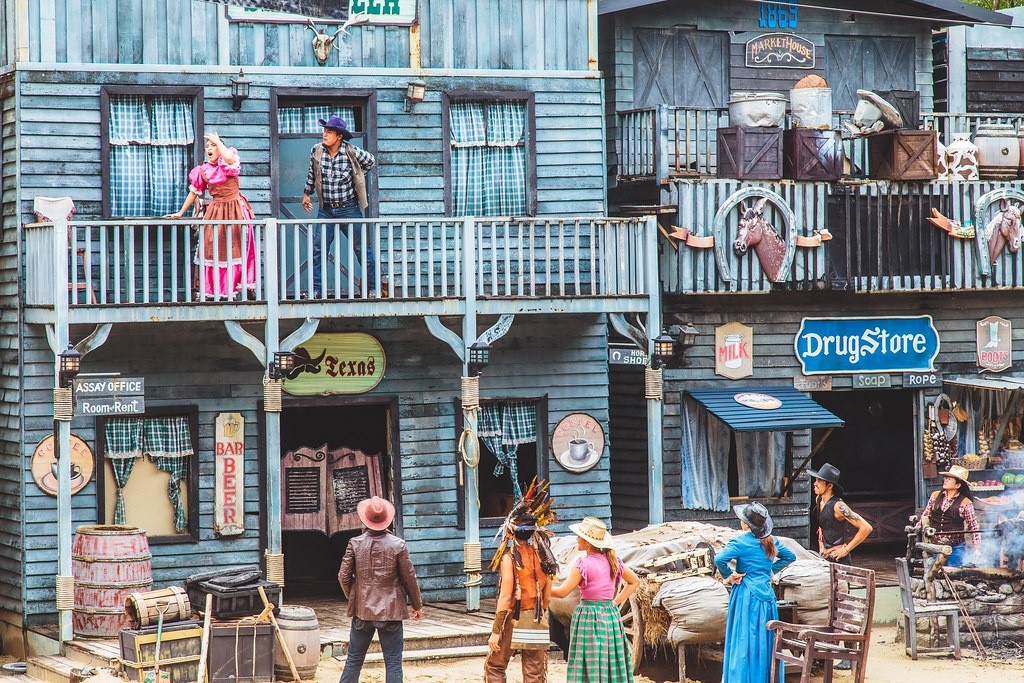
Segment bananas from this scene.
[952,402,969,422]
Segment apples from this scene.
[969,480,1003,486]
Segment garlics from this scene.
[924,422,993,471]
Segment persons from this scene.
[916,465,981,568]
[550,516,641,683]
[484,474,564,683]
[302,116,385,300]
[162,131,256,301]
[806,462,873,670]
[715,501,796,683]
[338,495,423,683]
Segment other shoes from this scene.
[835,658,852,670]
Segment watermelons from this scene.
[1002,472,1024,484]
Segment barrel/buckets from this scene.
[73,525,153,637]
[975,125,1024,181]
[726,92,788,128]
[937,132,979,181]
[790,88,832,129]
[274,605,319,682]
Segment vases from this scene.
[937,132,949,181]
[946,132,980,180]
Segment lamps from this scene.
[403,79,426,115]
[57,339,82,389]
[467,342,493,377]
[651,328,677,370]
[269,351,295,383]
[229,68,252,112]
[675,321,700,355]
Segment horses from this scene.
[986,198,1024,266]
[734,197,786,282]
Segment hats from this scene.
[356,494,395,531]
[319,115,353,142]
[733,501,773,538]
[938,465,972,486]
[805,463,844,494]
[568,516,613,549]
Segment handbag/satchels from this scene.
[510,610,551,650]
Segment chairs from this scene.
[895,557,962,661]
[33,196,98,305]
[764,563,876,683]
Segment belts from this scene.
[323,197,357,208]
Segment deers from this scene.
[305,10,370,63]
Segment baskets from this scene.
[960,455,987,471]
[972,495,1016,511]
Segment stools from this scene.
[643,542,718,583]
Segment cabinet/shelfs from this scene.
[769,580,803,675]
[846,499,915,544]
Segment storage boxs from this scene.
[782,129,845,182]
[198,621,276,683]
[872,89,921,130]
[868,129,939,182]
[184,580,281,620]
[118,620,208,683]
[716,126,784,182]
